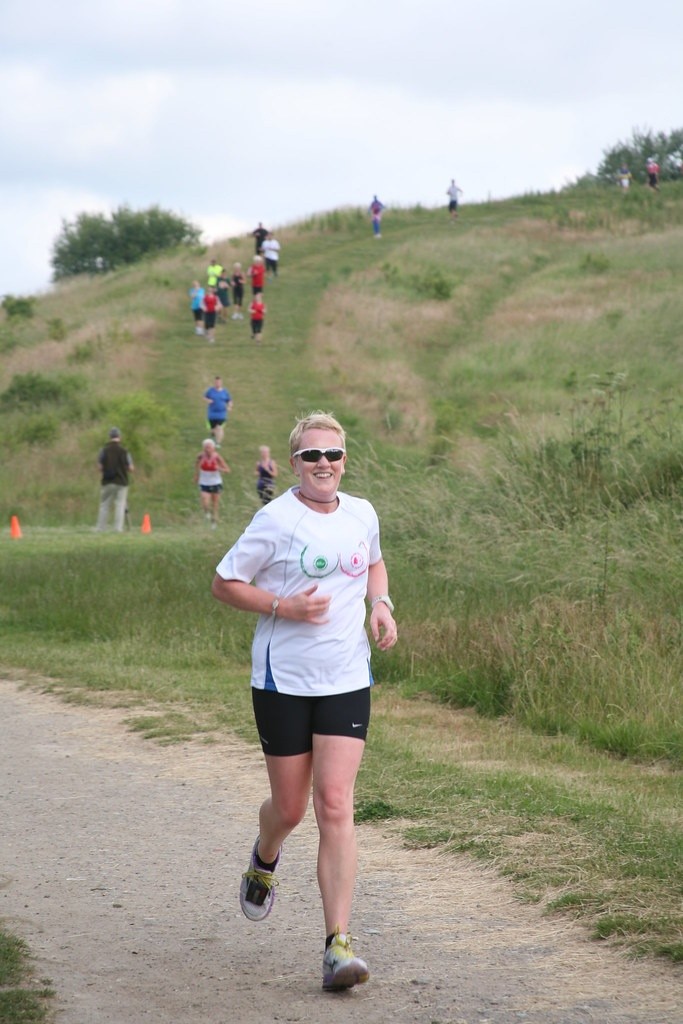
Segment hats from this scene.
[108,427,121,435]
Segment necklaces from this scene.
[298,490,341,505]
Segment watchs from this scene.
[372,595,394,614]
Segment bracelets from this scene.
[271,599,279,618]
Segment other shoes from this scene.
[214,443,222,449]
[267,272,279,283]
[204,510,219,531]
[206,334,216,343]
[195,327,205,336]
[231,312,244,320]
[93,526,124,534]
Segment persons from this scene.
[230,261,246,320]
[187,280,206,335]
[215,270,231,307]
[258,232,282,275]
[247,291,267,341]
[199,286,224,343]
[247,255,265,293]
[202,376,233,449]
[91,428,134,531]
[207,260,224,287]
[369,196,385,239]
[646,157,660,193]
[255,444,276,503]
[211,411,399,992]
[193,439,230,522]
[253,223,271,256]
[446,179,463,219]
[619,163,633,188]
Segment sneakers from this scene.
[320,923,369,991]
[239,835,283,921]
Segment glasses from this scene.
[291,447,347,462]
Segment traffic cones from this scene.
[9,516,23,539]
[140,514,152,535]
